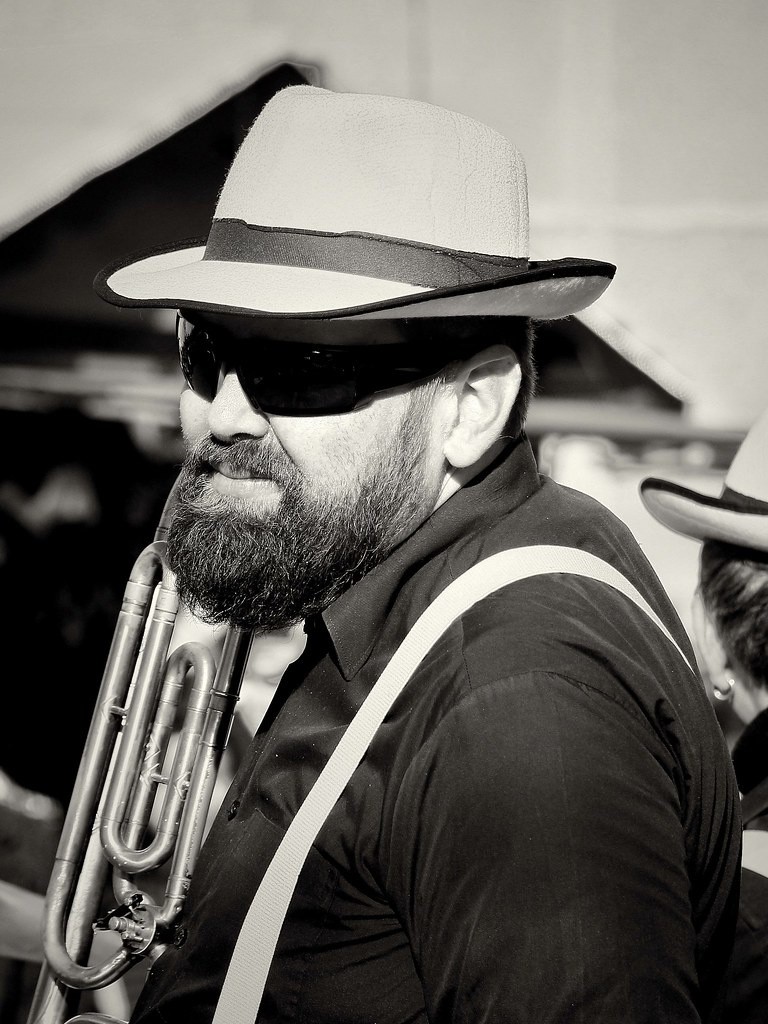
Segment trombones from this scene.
[22,464,258,1024]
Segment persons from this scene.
[638,410,768,1024]
[94,84,741,1023]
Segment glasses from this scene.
[173,312,496,417]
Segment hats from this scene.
[638,424,768,552]
[92,86,616,324]
